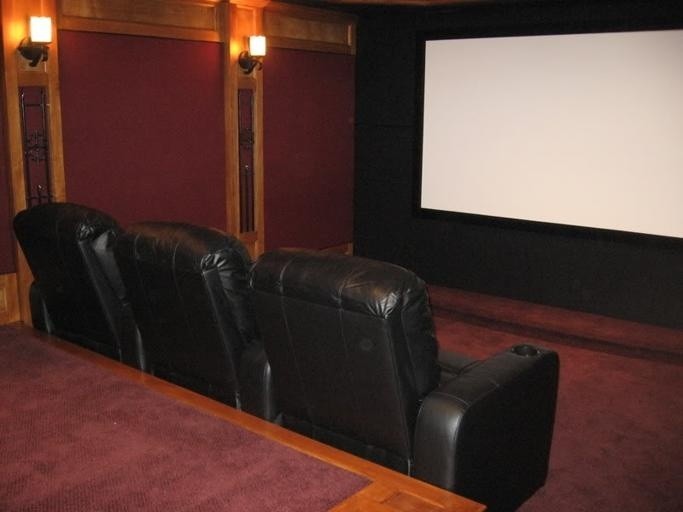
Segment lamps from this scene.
[239,35,266,75]
[16,16,51,66]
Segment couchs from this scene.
[13,204,558,511]
